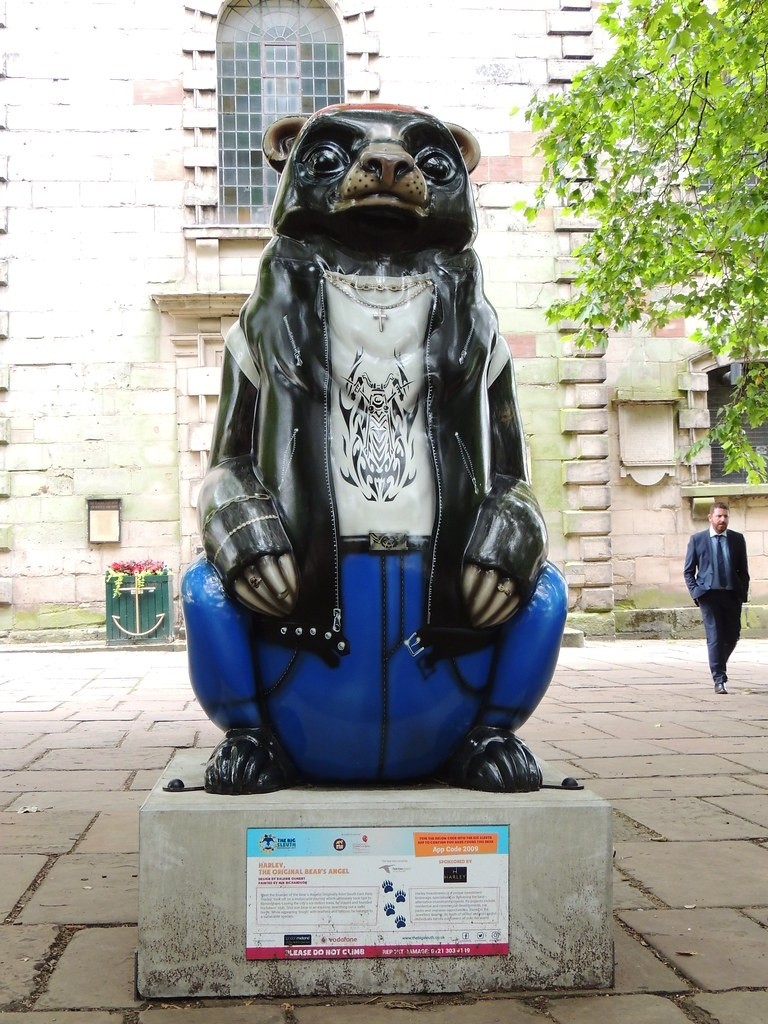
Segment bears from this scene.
[181,103,567,795]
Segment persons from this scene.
[683,502,750,694]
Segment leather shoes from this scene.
[723,676,728,682]
[715,683,727,694]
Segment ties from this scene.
[715,535,728,587]
